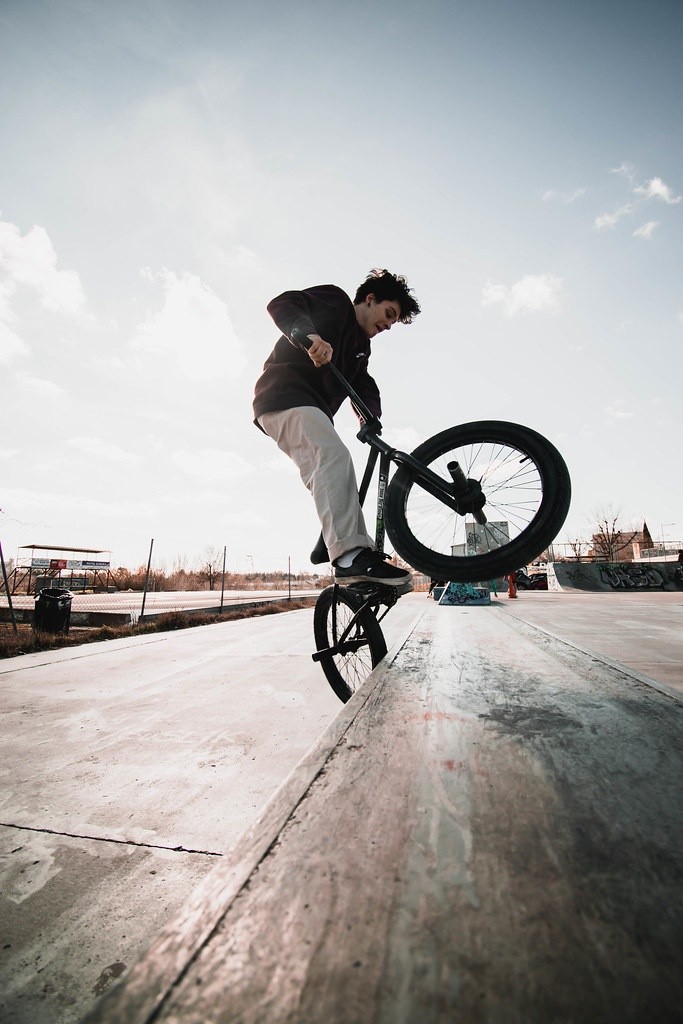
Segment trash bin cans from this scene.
[40,588,73,636]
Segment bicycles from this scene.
[290,327,572,705]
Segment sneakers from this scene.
[334,548,412,585]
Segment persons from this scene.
[251,266,422,598]
